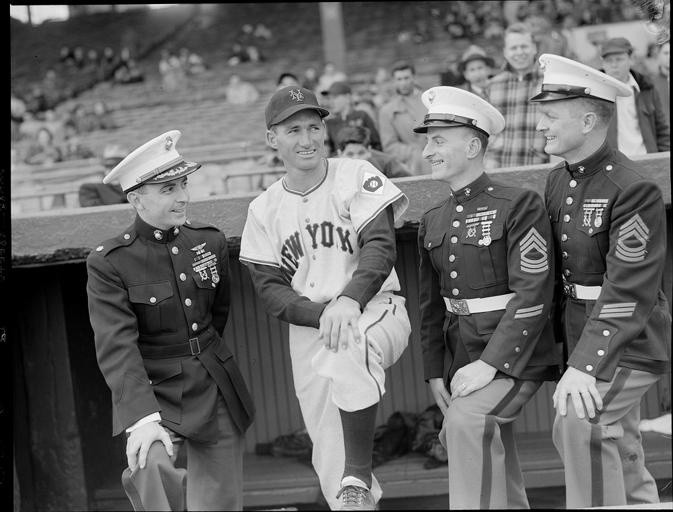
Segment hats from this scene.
[413,85,504,136]
[457,47,495,68]
[265,87,329,130]
[530,54,633,103]
[103,130,200,193]
[321,82,351,97]
[601,37,632,58]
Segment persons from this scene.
[85,129,256,512]
[10,17,352,210]
[411,84,555,510]
[323,1,671,179]
[239,85,412,510]
[529,51,673,508]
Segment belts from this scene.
[562,279,602,300]
[139,326,214,358]
[443,293,515,317]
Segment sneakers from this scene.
[338,486,376,511]
